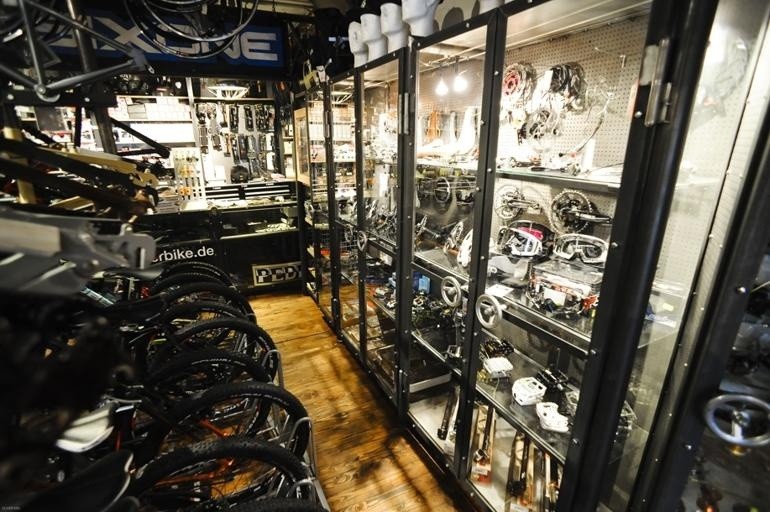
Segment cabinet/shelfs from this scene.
[12,56,306,295]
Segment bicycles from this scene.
[2,261,327,512]
[0,3,262,106]
